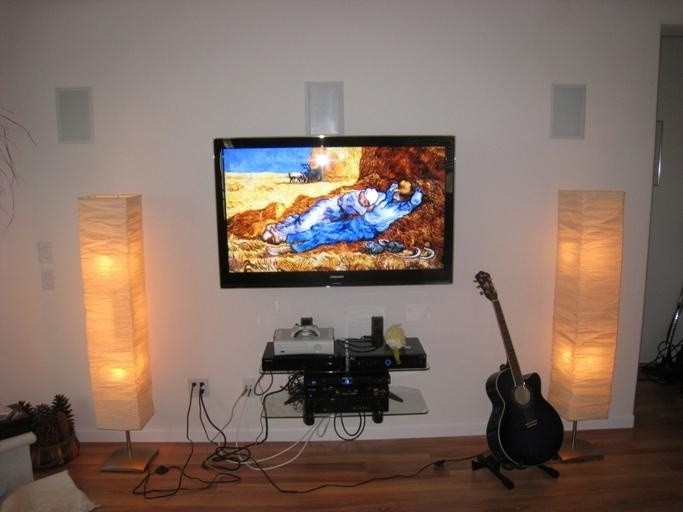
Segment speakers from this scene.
[371,316,383,348]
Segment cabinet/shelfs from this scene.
[259,364,430,419]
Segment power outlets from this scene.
[242,378,254,397]
[190,380,209,398]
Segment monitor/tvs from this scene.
[215,134,455,289]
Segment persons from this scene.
[265,177,427,257]
[263,186,378,242]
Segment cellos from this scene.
[473,271,564,470]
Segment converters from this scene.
[154,465,169,476]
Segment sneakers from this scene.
[362,240,386,255]
[378,237,405,253]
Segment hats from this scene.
[392,180,414,196]
[364,187,378,205]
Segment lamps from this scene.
[77,195,159,474]
[548,190,625,464]
